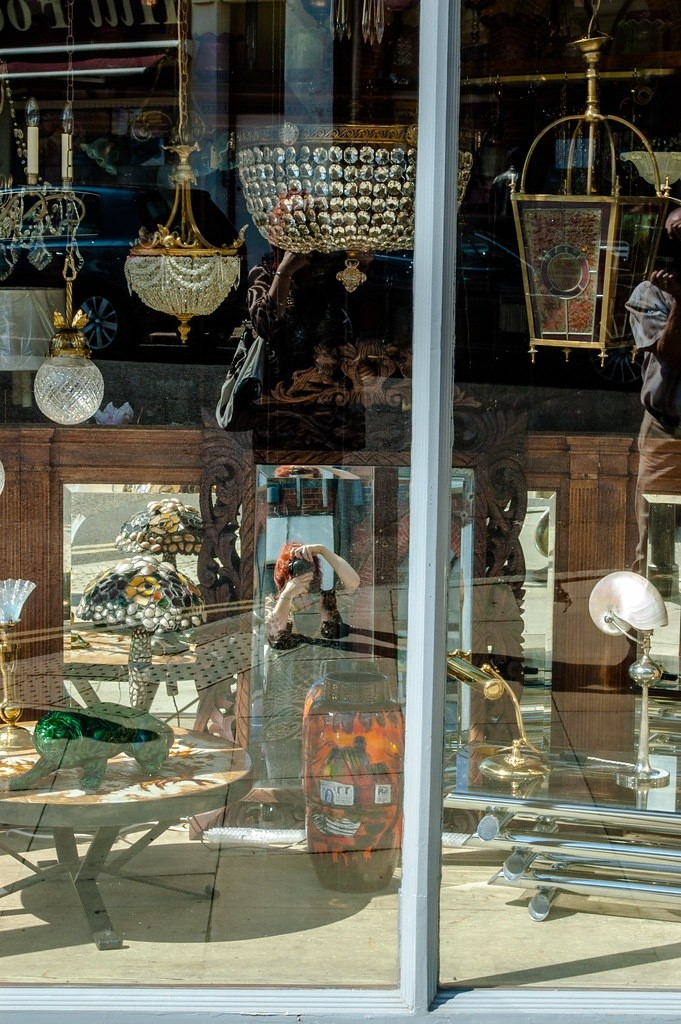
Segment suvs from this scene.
[1,182,248,361]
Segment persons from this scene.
[597,207,681,687]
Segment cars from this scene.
[371,227,546,339]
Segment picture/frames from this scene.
[65,485,244,741]
[518,491,556,751]
[249,464,475,789]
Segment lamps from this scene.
[125,0,248,345]
[238,0,472,294]
[0,579,38,749]
[444,651,551,778]
[589,572,672,788]
[508,0,669,367]
[33,1,104,426]
[0,60,86,282]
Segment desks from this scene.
[0,719,254,950]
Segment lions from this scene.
[8,701,174,794]
